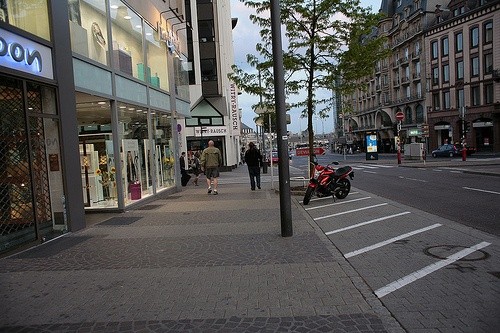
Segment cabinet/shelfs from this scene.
[151,76,160,87]
[69,20,89,57]
[80,150,104,203]
[136,62,150,83]
[106,49,132,76]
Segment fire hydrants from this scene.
[461,148,467,161]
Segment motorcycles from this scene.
[304,154,355,204]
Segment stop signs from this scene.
[396,112,404,121]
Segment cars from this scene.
[296,141,329,148]
[267,150,279,166]
[288,152,292,160]
[432,144,477,158]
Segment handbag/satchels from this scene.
[191,159,196,168]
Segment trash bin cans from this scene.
[308,153,318,179]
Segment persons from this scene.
[201,140,222,195]
[126,151,135,183]
[180,152,186,177]
[245,142,261,191]
[240,144,246,153]
[191,152,201,186]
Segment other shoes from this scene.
[195,184,199,186]
[192,181,196,185]
[214,190,218,195]
[257,184,261,189]
[208,188,212,193]
[251,188,255,191]
